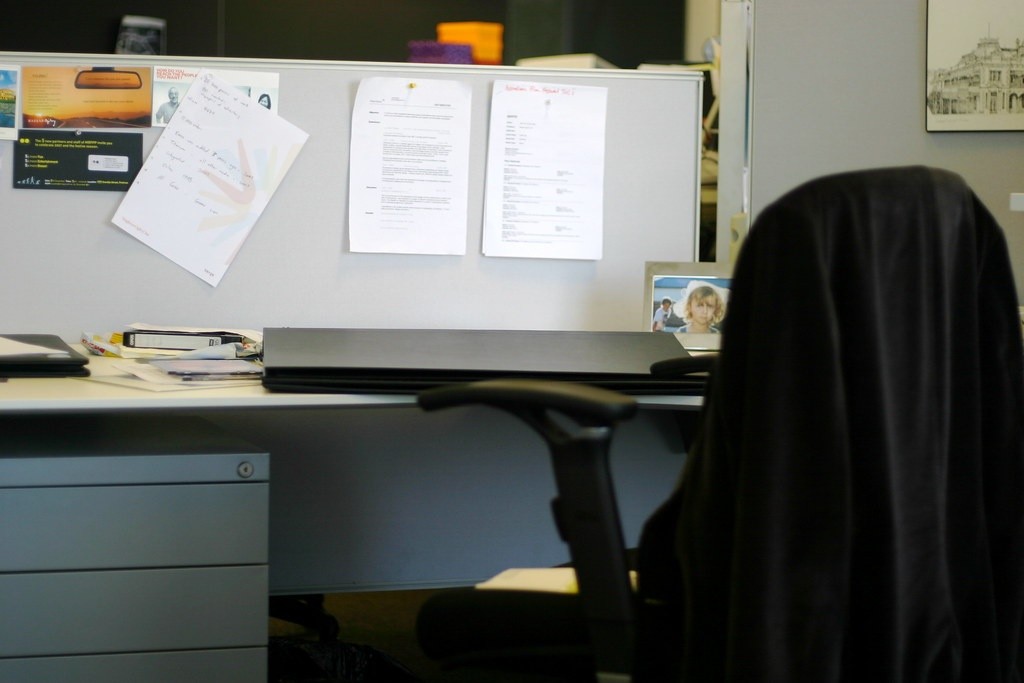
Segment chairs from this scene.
[414,166,1024,683]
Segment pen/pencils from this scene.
[181,374,263,381]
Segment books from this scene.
[81,324,263,382]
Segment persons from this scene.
[653,299,671,331]
[673,280,727,334]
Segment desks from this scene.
[0,327,721,410]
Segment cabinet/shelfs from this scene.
[0,414,270,683]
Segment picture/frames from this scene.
[925,0,1024,134]
